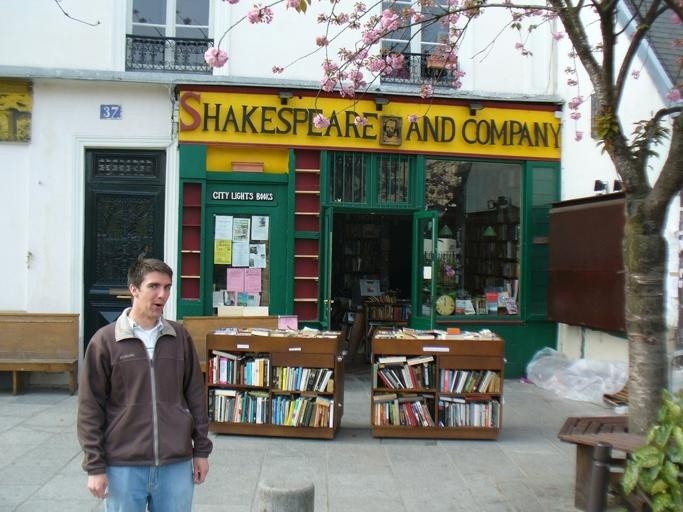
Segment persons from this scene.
[75,254,212,511]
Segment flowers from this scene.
[431,251,462,293]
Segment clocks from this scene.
[434,295,455,316]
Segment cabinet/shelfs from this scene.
[331,212,410,364]
[205,330,343,440]
[463,204,518,301]
[370,327,503,441]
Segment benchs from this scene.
[0,311,80,396]
[557,417,647,512]
[182,315,298,374]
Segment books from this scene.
[207,326,343,429]
[372,328,501,429]
[334,214,520,358]
